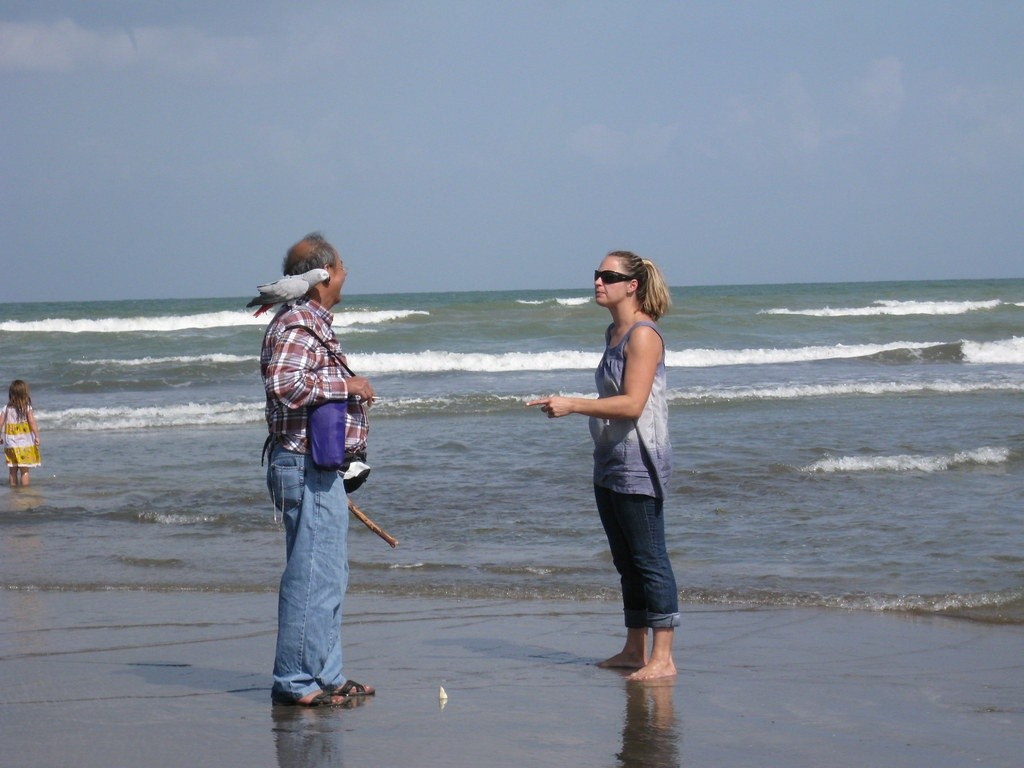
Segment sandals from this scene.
[330,679,376,697]
[271,687,352,707]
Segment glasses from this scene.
[594,270,640,283]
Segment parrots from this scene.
[246,268,330,318]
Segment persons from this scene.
[1,379,41,486]
[262,236,378,709]
[522,251,680,683]
